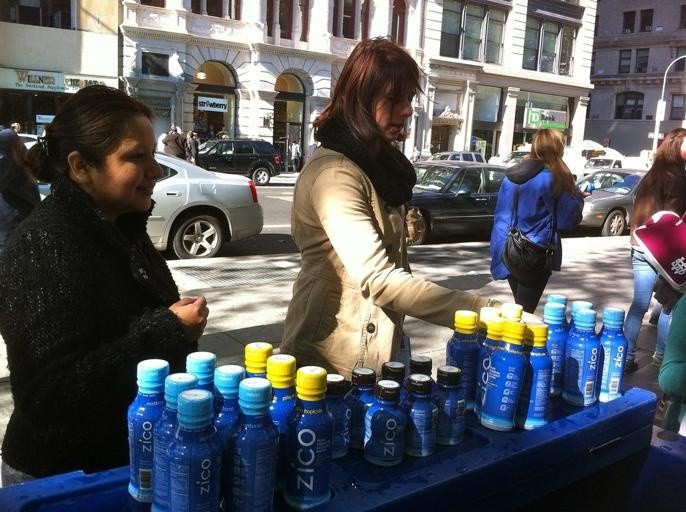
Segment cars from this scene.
[574,168,650,236]
[425,151,488,166]
[0,132,266,260]
[196,141,282,187]
[499,150,570,168]
[406,161,508,246]
[3,129,45,161]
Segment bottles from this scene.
[283,365,335,507]
[482,318,510,398]
[342,369,375,459]
[478,323,526,430]
[366,379,408,466]
[184,352,220,418]
[382,363,409,410]
[400,373,437,457]
[125,358,169,502]
[502,304,523,328]
[211,363,247,439]
[543,296,570,396]
[263,355,300,431]
[597,307,628,402]
[567,302,595,335]
[547,294,568,330]
[244,342,272,381]
[480,308,502,341]
[227,378,279,511]
[562,301,602,405]
[169,390,222,511]
[433,365,466,445]
[408,356,437,398]
[514,323,553,430]
[325,373,350,459]
[445,310,481,410]
[148,373,197,512]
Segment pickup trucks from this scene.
[582,159,624,180]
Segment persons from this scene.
[490,129,585,313]
[409,146,421,163]
[659,276,686,433]
[289,141,303,172]
[489,154,503,166]
[0,84,207,479]
[186,130,200,167]
[0,129,42,240]
[164,125,187,159]
[216,123,230,140]
[156,131,170,153]
[278,39,546,383]
[622,128,685,372]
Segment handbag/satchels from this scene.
[635,204,686,292]
[501,162,557,282]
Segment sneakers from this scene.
[649,317,659,327]
[624,361,638,375]
[652,353,665,366]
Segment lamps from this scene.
[198,66,206,81]
